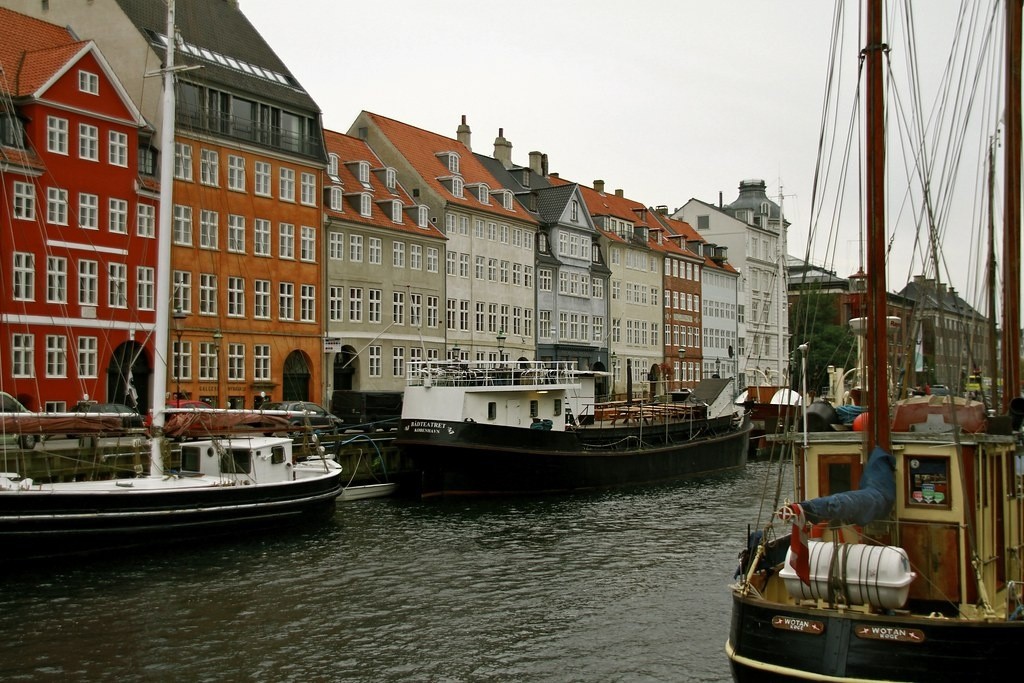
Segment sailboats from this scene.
[0,0,344,572]
[715,1,1024,682]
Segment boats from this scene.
[391,353,756,503]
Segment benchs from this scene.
[609,410,701,426]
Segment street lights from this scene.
[213,328,223,411]
[171,306,188,411]
[496,327,507,368]
[611,352,618,407]
[678,346,686,391]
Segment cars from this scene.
[258,400,343,438]
[145,399,227,442]
[65,403,143,438]
[0,391,44,450]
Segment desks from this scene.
[658,403,692,421]
[604,409,648,426]
[620,406,664,425]
[643,405,680,424]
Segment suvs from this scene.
[331,390,411,434]
